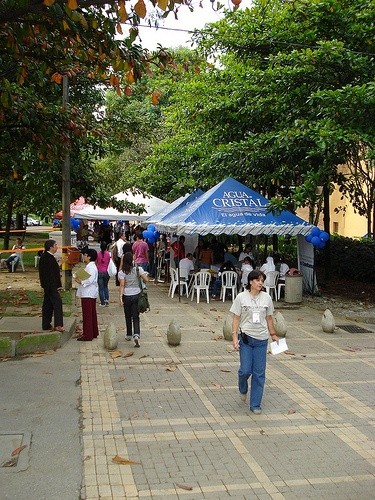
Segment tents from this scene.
[157,176,318,304]
[142,187,225,299]
[54,176,171,285]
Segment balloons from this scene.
[318,229,325,238]
[305,234,314,242]
[319,231,329,242]
[311,227,320,237]
[317,238,326,248]
[311,236,320,246]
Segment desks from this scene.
[197,264,243,295]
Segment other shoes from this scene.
[99,304,106,308]
[240,393,247,401]
[77,336,92,341]
[105,299,110,305]
[125,333,132,341]
[253,409,262,415]
[54,325,65,332]
[134,336,140,347]
[210,294,217,301]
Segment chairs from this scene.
[278,283,286,301]
[8,257,25,273]
[262,271,279,302]
[167,267,189,299]
[190,271,212,304]
[34,255,41,268]
[219,270,238,304]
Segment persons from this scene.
[229,269,280,415]
[95,241,111,308]
[74,248,100,342]
[5,237,26,273]
[39,239,67,332]
[110,221,298,301]
[117,252,149,348]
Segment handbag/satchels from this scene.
[137,292,149,313]
[106,252,117,276]
[111,245,118,262]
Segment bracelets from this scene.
[231,331,238,336]
[270,332,277,336]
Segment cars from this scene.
[12,215,39,226]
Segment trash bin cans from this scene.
[285,268,303,304]
